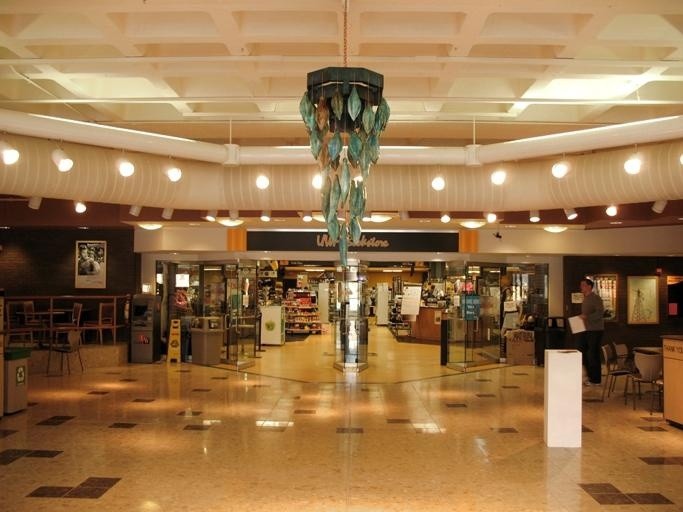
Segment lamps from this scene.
[0,300,116,377]
[543,314,663,417]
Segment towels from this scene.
[181,320,189,363]
[4,348,31,413]
[534,316,568,366]
[190,316,225,365]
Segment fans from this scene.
[400,286,421,315]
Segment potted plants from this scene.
[587,273,620,323]
[624,275,660,326]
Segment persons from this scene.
[575,279,605,386]
[78,245,100,275]
[500,288,523,359]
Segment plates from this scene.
[281,300,324,336]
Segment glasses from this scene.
[300,1,388,273]
[0,106,682,228]
